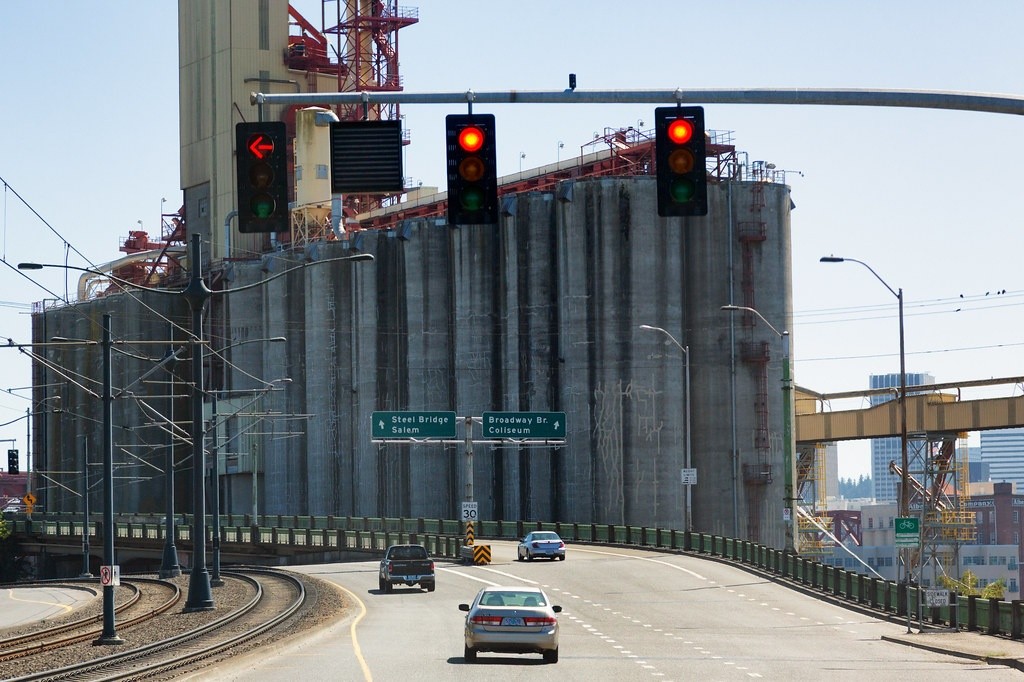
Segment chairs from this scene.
[486,596,501,605]
[524,596,537,606]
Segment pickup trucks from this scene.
[380,545,436,593]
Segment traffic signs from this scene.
[482,411,568,439]
[370,410,457,438]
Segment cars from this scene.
[518,531,566,562]
[458,587,562,663]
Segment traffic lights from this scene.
[655,106,708,216]
[8,450,20,475]
[446,114,500,225]
[235,122,289,233]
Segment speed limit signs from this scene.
[462,501,479,522]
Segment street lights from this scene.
[18,231,375,614]
[720,303,798,556]
[820,256,912,582]
[639,325,693,551]
[75,434,94,577]
[26,396,61,520]
